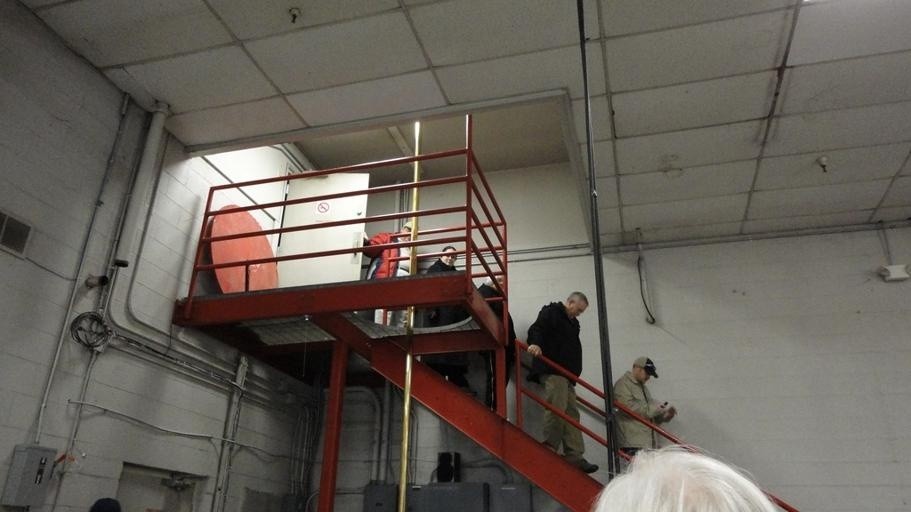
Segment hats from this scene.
[633,356,659,378]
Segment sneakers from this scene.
[574,459,598,473]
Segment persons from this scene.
[475,271,517,410]
[525,290,600,474]
[585,444,782,512]
[363,221,412,327]
[613,356,679,450]
[423,244,472,390]
